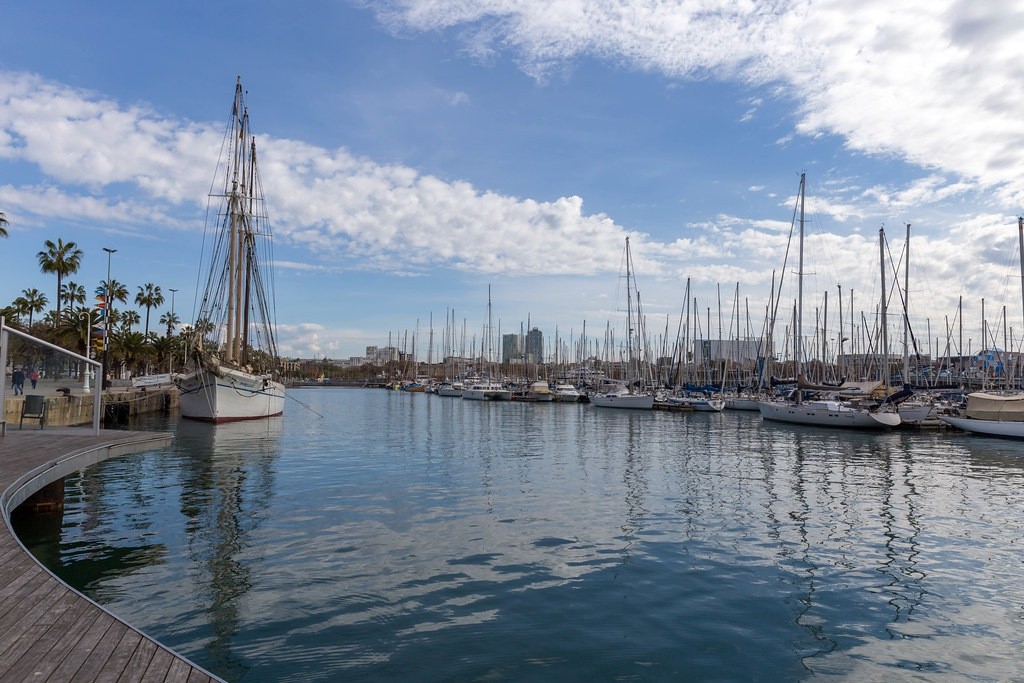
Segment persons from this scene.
[11,368,26,396]
[737,384,741,398]
[228,359,253,374]
[30,368,37,389]
[728,383,732,392]
[106,373,110,382]
[669,375,673,386]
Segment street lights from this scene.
[76,312,96,394]
[102,247,117,389]
[168,288,179,374]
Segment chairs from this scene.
[20,395,46,429]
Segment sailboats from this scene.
[182,74,290,425]
[408,176,1023,447]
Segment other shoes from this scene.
[14,393,18,396]
[20,391,24,395]
